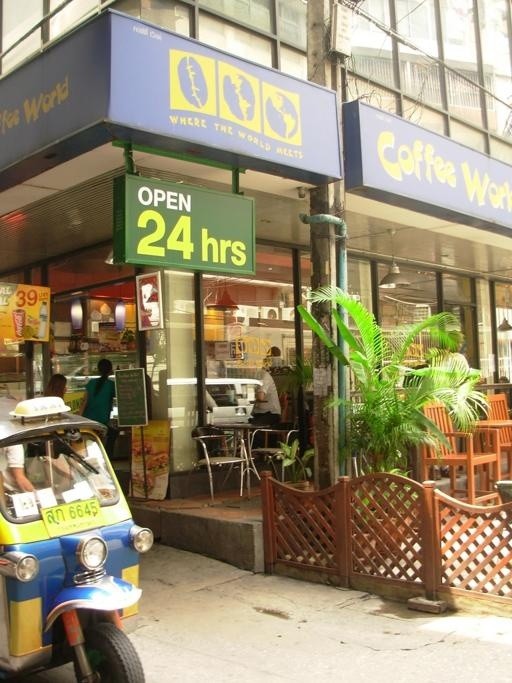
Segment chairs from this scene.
[467,393,512,480]
[184,426,247,501]
[417,398,502,508]
[249,422,300,482]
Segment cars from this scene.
[64,365,116,387]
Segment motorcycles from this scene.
[0,395,154,683]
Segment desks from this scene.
[208,422,271,490]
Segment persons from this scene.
[205,388,239,469]
[240,357,282,469]
[57,427,105,484]
[77,357,116,423]
[0,442,35,494]
[44,373,68,401]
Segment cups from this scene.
[13,310,26,339]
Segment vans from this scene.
[165,378,263,427]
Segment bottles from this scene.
[38,300,49,339]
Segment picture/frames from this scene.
[136,272,165,332]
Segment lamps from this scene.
[69,297,83,332]
[498,295,511,332]
[377,228,411,288]
[112,299,128,332]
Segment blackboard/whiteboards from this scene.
[115,368,148,427]
